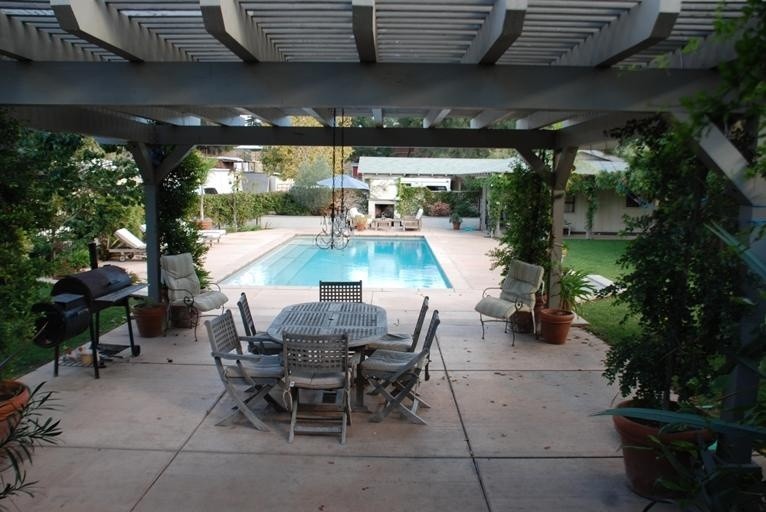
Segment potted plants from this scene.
[149,214,217,330]
[448,211,463,229]
[487,158,553,333]
[596,112,751,500]
[130,295,165,338]
[1,217,58,448]
[539,264,596,344]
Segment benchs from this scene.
[199,229,226,246]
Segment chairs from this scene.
[107,226,146,262]
[476,259,547,347]
[203,278,438,446]
[159,251,229,343]
[370,214,422,233]
